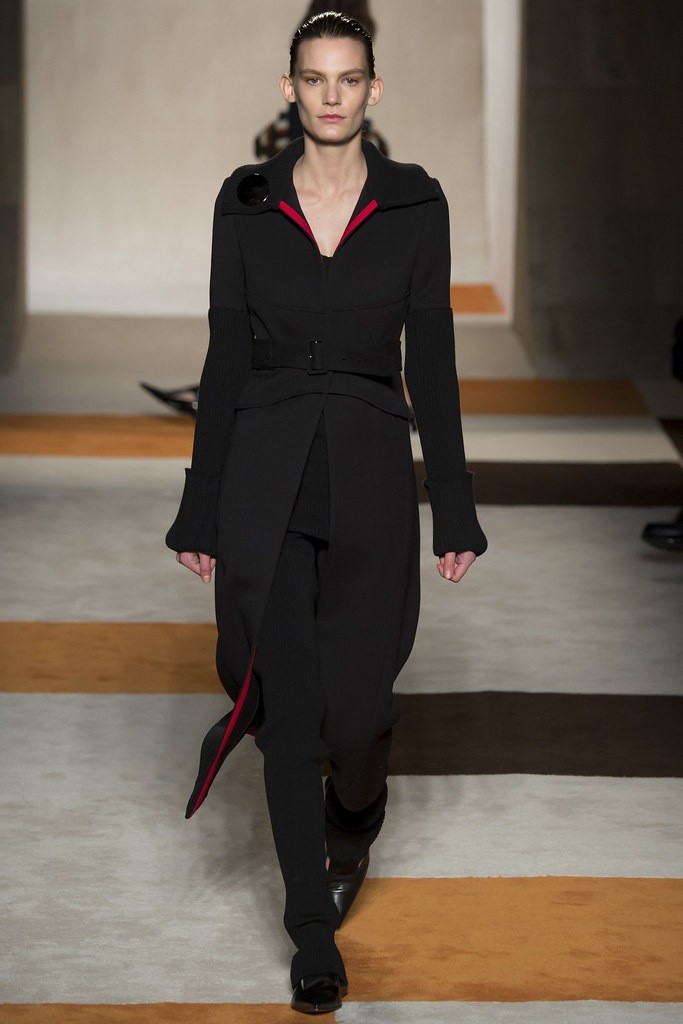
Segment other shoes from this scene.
[640,520,683,551]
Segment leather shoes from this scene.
[326,847,371,928]
[290,975,348,1015]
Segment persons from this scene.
[168,11,487,1013]
